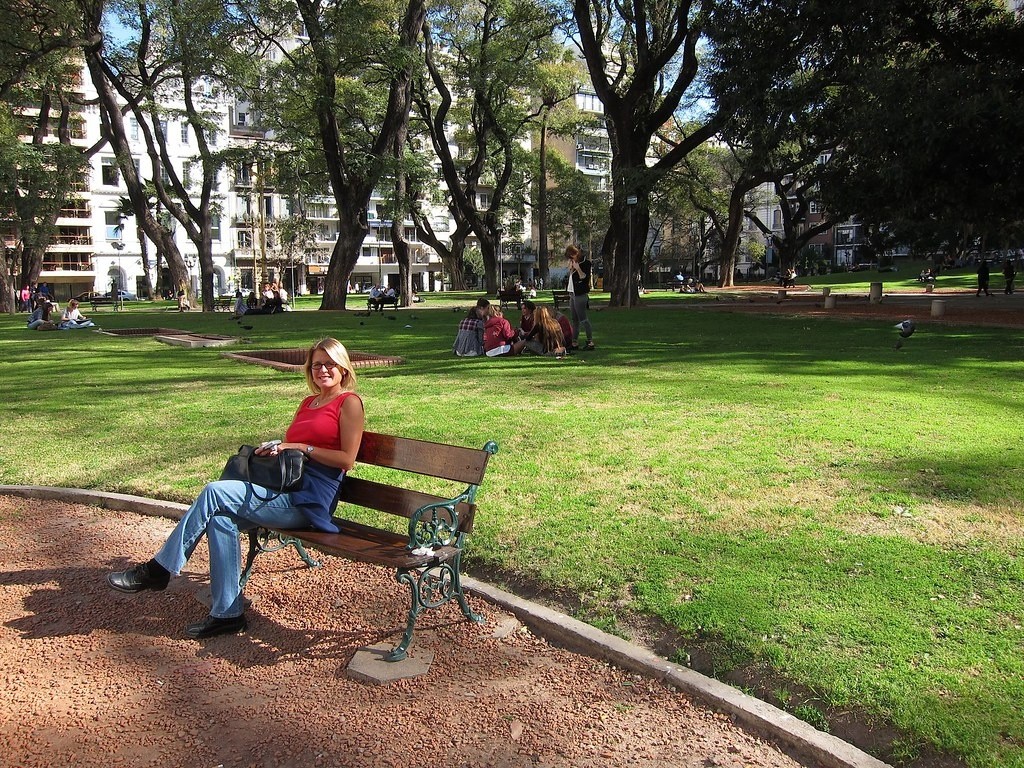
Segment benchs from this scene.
[238,431,498,662]
[211,294,233,312]
[551,290,590,311]
[366,293,400,311]
[497,289,524,311]
[665,279,699,292]
[89,297,119,312]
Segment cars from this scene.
[232,288,251,299]
[92,289,139,301]
[66,291,102,302]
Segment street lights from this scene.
[110,241,124,297]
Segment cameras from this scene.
[258,439,281,451]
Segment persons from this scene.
[168,290,174,299]
[28,302,55,329]
[1002,260,1017,294]
[916,268,935,283]
[667,271,707,294]
[178,280,190,312]
[19,280,53,314]
[368,282,396,313]
[58,299,92,329]
[453,298,516,358]
[499,277,537,299]
[976,260,991,297]
[783,269,797,289]
[107,336,365,640]
[512,301,573,356]
[234,281,288,315]
[565,246,597,351]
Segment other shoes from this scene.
[567,343,578,350]
[582,340,595,350]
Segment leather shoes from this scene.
[184,612,248,638]
[106,560,171,593]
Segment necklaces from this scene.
[314,389,342,406]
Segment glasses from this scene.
[311,361,338,369]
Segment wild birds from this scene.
[229,315,242,320]
[716,296,720,302]
[384,316,396,320]
[452,307,461,312]
[596,308,602,311]
[237,317,242,324]
[894,318,915,338]
[893,339,901,350]
[360,321,363,325]
[405,324,412,329]
[411,314,418,319]
[240,326,254,330]
[353,311,372,317]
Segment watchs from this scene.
[305,444,315,455]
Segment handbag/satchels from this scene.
[218,444,309,500]
